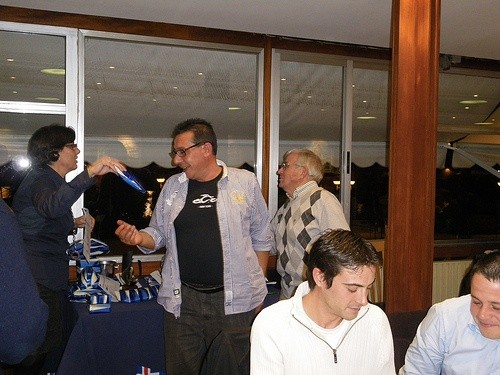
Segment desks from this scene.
[55,283,281,375]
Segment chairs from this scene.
[388,311,428,375]
[201,327,250,375]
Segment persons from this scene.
[249,229,397,375]
[115,118,273,375]
[398,250,500,375]
[270,148,351,301]
[0,198,50,375]
[11,124,127,375]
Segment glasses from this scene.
[168,142,202,158]
[63,143,79,148]
[281,161,305,170]
